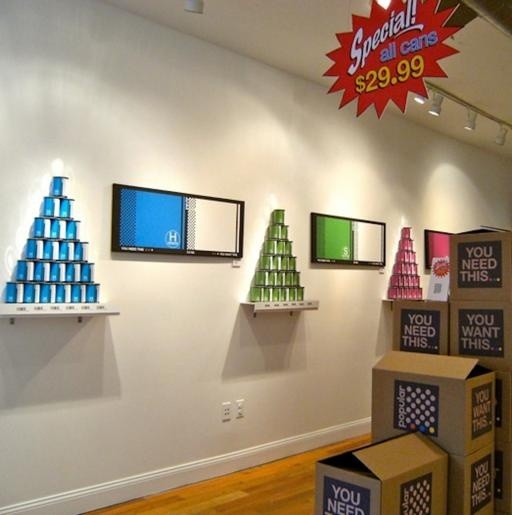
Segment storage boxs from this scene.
[392,299,449,355]
[449,225,512,301]
[315,431,449,515]
[371,441,494,515]
[372,350,496,457]
[495,371,512,442]
[450,301,512,371]
[494,440,512,513]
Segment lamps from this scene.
[415,81,512,146]
[184,0,203,14]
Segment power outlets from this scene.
[220,402,232,421]
